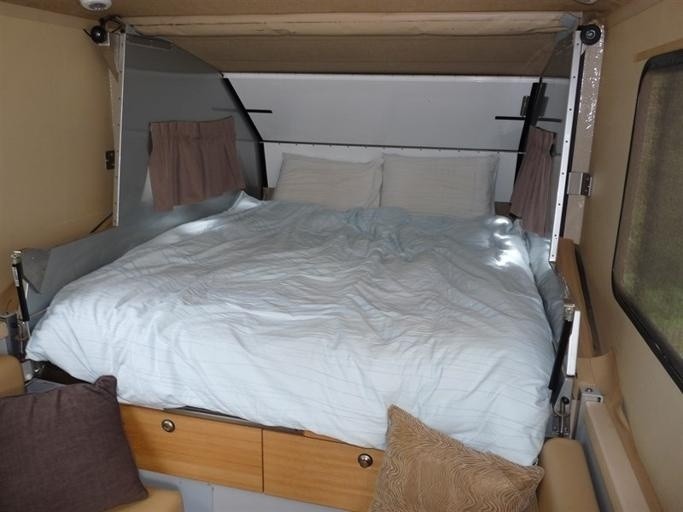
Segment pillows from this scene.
[0,376,149,512]
[269,151,382,211]
[379,155,498,221]
[367,405,546,512]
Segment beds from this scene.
[9,0,607,511]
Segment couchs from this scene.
[531,437,604,512]
[0,353,182,512]
